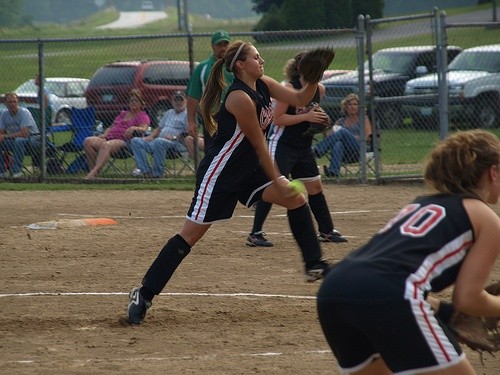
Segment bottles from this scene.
[145,127,152,135]
[96,122,103,133]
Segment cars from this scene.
[0,78,93,125]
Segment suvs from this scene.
[86,58,206,128]
[321,47,462,125]
[404,45,500,127]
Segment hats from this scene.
[210,29,232,43]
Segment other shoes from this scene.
[312,147,336,178]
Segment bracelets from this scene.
[173,136,178,140]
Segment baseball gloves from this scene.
[298,48,335,83]
[298,102,331,134]
[449,283,500,354]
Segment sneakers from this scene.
[304,263,330,277]
[127,287,151,325]
[245,231,274,247]
[317,229,347,243]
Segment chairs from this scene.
[103,134,143,176]
[47,105,98,165]
[163,146,194,176]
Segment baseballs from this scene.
[288,179,305,197]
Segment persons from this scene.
[83,88,149,181]
[0,92,41,178]
[34,73,56,114]
[131,89,194,179]
[316,128,500,374]
[128,39,338,325]
[245,51,349,247]
[186,30,235,148]
[311,92,369,178]
